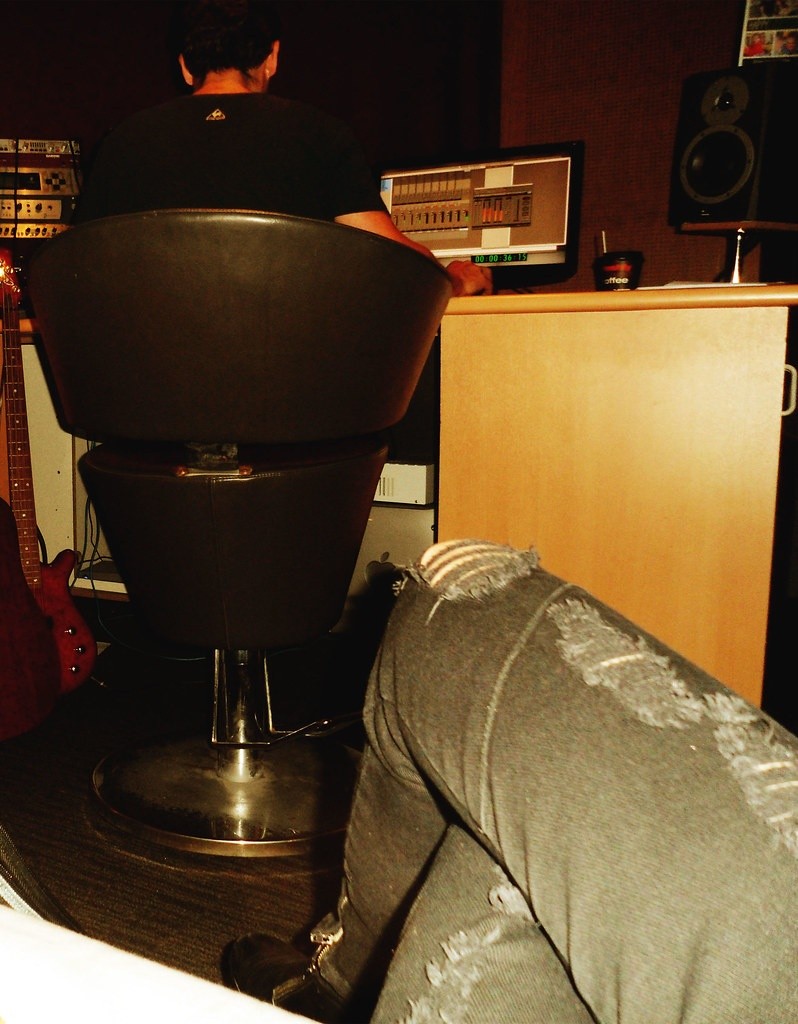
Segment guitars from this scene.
[0,494,64,741]
[0,247,98,697]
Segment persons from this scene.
[75,0,493,298]
[219,538,798,1023]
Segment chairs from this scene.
[24,208,461,877]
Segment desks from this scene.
[19,283,798,714]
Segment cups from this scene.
[603,250,645,291]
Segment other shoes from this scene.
[223,933,369,1024]
